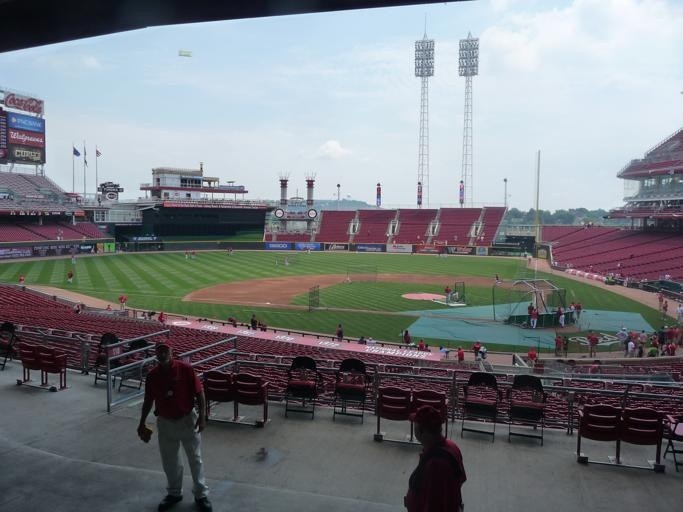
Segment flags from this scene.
[97,150,102,157]
[74,147,80,156]
[84,148,87,166]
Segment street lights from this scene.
[458,31,479,208]
[414,13,434,211]
[336,184,339,211]
[503,178,507,207]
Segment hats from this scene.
[408,404,443,424]
[154,338,172,348]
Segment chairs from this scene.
[615,160,683,178]
[263,207,507,247]
[572,360,683,475]
[151,319,583,446]
[541,207,683,286]
[0,282,151,395]
[0,172,106,244]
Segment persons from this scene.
[228,317,237,327]
[251,314,257,330]
[228,248,232,256]
[106,305,112,311]
[18,273,25,285]
[184,248,189,261]
[358,336,365,344]
[119,295,127,310]
[306,246,310,254]
[72,251,76,264]
[137,340,212,512]
[74,301,81,317]
[336,324,343,342]
[158,312,165,323]
[67,271,73,283]
[192,247,196,259]
[404,405,466,512]
[404,287,683,363]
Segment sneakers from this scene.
[194,494,212,511]
[157,494,182,511]
